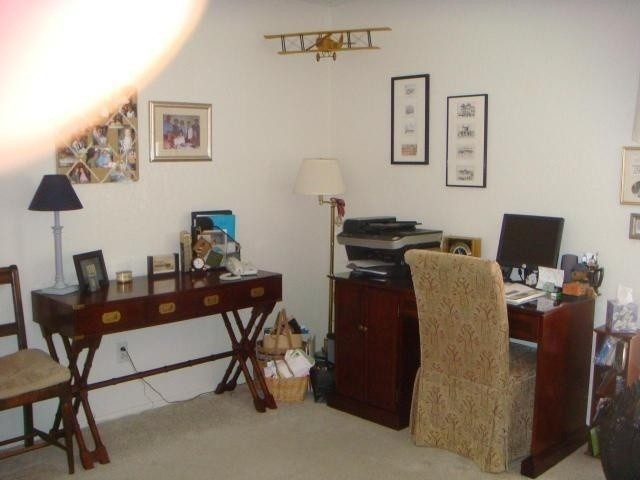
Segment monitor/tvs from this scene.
[496,213,566,288]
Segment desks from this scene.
[32,264,283,469]
[326,270,593,476]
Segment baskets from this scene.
[263,310,303,355]
[261,360,310,401]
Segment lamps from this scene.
[28,174,85,295]
[291,158,344,360]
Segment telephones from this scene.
[225,257,258,275]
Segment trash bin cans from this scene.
[310,361,333,402]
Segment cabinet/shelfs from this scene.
[585,323,640,460]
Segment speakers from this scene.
[561,254,578,284]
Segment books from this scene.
[504,280,548,306]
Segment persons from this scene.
[163,114,200,150]
[56,88,137,184]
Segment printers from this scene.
[336,216,443,279]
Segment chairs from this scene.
[0,265,76,476]
[404,250,536,476]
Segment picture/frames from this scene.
[620,144,640,206]
[390,73,430,165]
[72,250,109,293]
[149,101,213,162]
[446,94,487,188]
